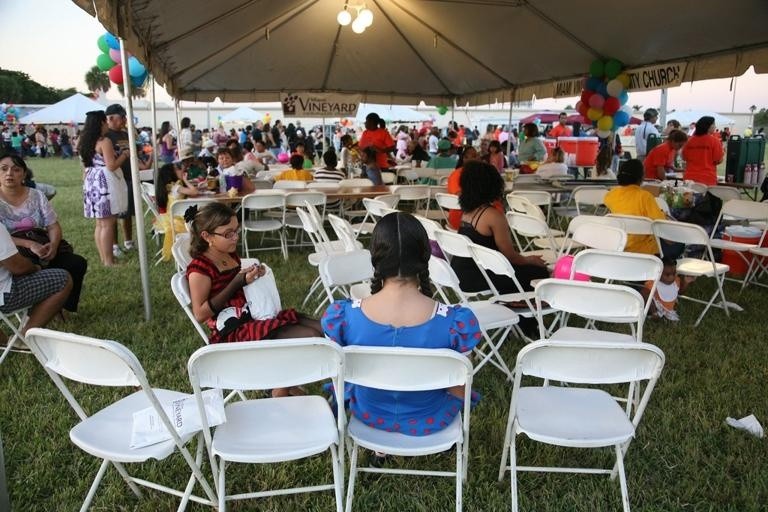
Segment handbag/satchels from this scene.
[241,172,255,196]
[10,227,73,258]
[215,305,248,336]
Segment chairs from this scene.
[185,336,347,512]
[1,155,768,422]
[497,340,666,511]
[26,328,220,512]
[338,345,474,512]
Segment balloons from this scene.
[2,103,20,126]
[95,30,149,90]
[575,58,631,137]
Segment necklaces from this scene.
[207,251,230,266]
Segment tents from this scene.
[519,113,566,138]
[222,107,262,124]
[567,114,595,128]
[479,117,524,153]
[18,93,110,145]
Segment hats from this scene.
[106,104,125,116]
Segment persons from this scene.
[634,108,767,264]
[138,116,346,265]
[332,112,632,227]
[76,111,130,270]
[638,257,686,323]
[105,103,139,258]
[0,152,89,324]
[2,124,81,160]
[186,202,326,401]
[319,210,482,472]
[449,159,553,343]
[603,157,686,266]
[0,221,75,352]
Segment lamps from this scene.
[337,2,374,33]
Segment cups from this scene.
[210,169,220,193]
[262,157,269,165]
[505,170,513,182]
[727,175,735,182]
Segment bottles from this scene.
[659,184,671,206]
[751,164,758,184]
[758,162,766,184]
[674,192,683,208]
[207,164,215,190]
[743,164,751,184]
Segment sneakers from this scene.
[113,245,122,257]
[124,241,134,249]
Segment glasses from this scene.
[209,225,241,239]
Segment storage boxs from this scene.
[540,137,556,160]
[725,134,766,185]
[558,135,599,166]
[645,134,664,154]
